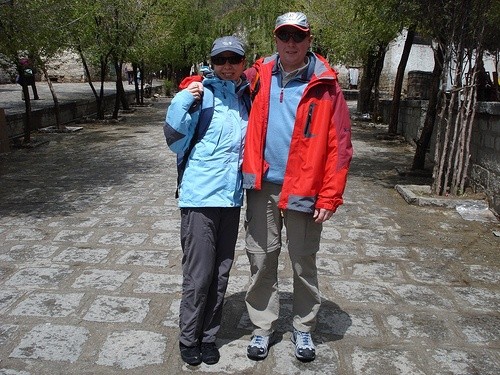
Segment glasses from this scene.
[209,54,245,65]
[275,28,309,43]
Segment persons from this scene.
[165,35,252,365]
[124,62,134,85]
[17,54,40,101]
[164,12,353,366]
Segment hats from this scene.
[210,35,247,57]
[273,11,312,33]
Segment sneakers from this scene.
[246,334,270,360]
[290,327,317,360]
[179,340,203,366]
[200,342,221,365]
